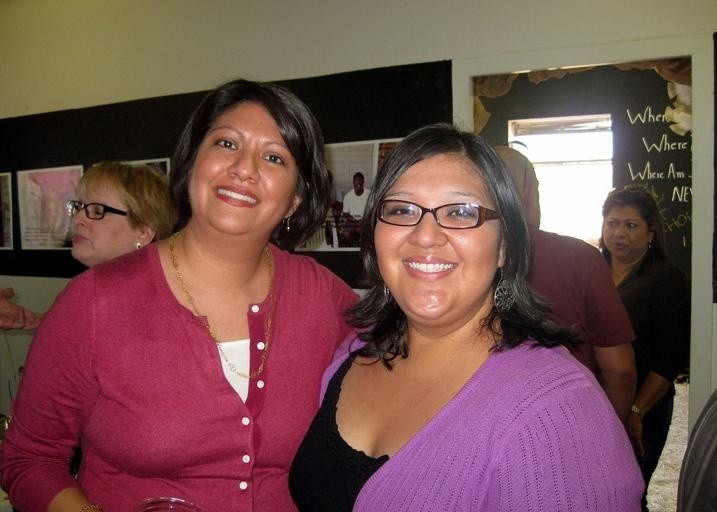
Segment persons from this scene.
[286,120,646,512]
[0,287,45,331]
[0,76,367,511]
[492,142,636,423]
[69,160,181,476]
[340,172,373,240]
[677,391,717,511]
[325,169,344,241]
[595,185,690,511]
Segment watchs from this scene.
[631,405,645,418]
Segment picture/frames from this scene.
[292,137,406,252]
[0,157,171,250]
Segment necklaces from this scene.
[168,229,272,378]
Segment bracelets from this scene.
[82,503,102,511]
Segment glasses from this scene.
[63,200,127,220]
[375,199,500,229]
[607,185,646,194]
